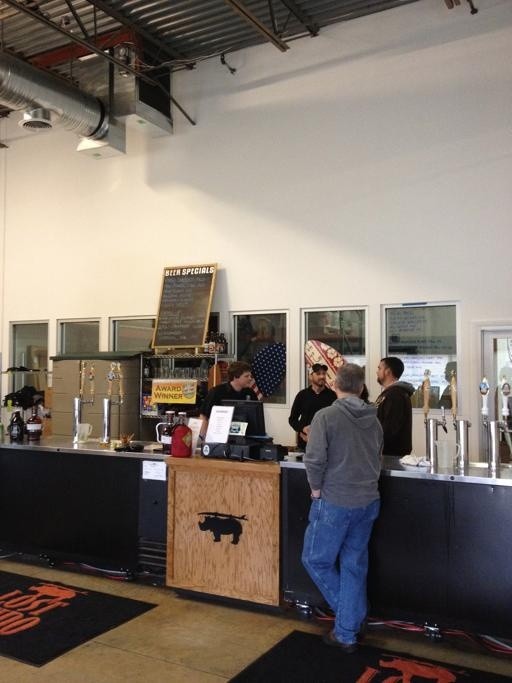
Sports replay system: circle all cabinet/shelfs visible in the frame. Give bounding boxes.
[49,358,161,443]
[139,350,234,443]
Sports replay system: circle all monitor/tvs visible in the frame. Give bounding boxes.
[221,400,266,446]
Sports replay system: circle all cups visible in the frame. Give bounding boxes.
[121,432,130,445]
[100,436,110,449]
[0,422,4,442]
[152,366,199,378]
[78,423,93,443]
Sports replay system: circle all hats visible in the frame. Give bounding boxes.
[309,363,327,374]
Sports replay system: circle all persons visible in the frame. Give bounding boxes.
[438,361,458,409]
[300,363,385,655]
[197,362,260,445]
[288,364,338,453]
[373,357,415,463]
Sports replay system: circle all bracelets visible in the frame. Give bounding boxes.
[198,434,204,442]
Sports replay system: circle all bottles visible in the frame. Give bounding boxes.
[203,329,227,354]
[200,358,208,377]
[41,412,52,437]
[27,399,42,442]
[197,413,208,447]
[9,409,24,443]
[162,409,177,454]
[170,411,192,458]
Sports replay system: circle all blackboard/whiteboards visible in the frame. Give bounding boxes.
[151,263,218,349]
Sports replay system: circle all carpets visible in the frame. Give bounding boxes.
[222,625,511,681]
[0,568,158,669]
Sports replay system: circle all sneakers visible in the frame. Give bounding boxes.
[324,631,358,654]
